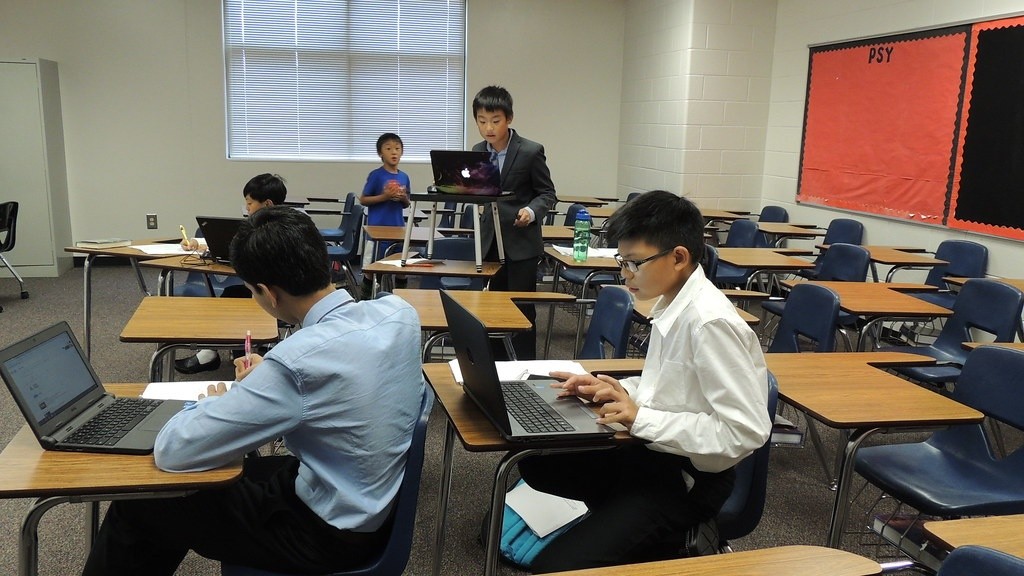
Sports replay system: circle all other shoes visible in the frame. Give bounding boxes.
[175,354,220,374]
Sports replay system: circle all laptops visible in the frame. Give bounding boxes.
[0,322,198,454]
[439,288,617,443]
[430,149,515,197]
[196,216,247,266]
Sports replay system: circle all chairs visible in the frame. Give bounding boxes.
[0,196,1024,574]
[0,200,28,313]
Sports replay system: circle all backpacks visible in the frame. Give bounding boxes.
[476,477,589,570]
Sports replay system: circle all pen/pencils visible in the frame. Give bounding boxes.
[244,330,251,370]
[406,264,434,267]
[180,225,190,246]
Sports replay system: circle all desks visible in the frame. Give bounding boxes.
[0,380,246,570]
[63,240,195,363]
[753,221,828,248]
[400,192,505,272]
[361,251,504,300]
[712,247,818,292]
[118,295,277,381]
[542,246,630,357]
[390,289,577,361]
[776,276,953,348]
[420,357,651,570]
[360,224,475,262]
[585,206,619,227]
[540,224,603,247]
[815,241,948,284]
[552,195,616,224]
[752,351,990,548]
[137,253,245,297]
[939,277,1024,344]
[690,205,754,243]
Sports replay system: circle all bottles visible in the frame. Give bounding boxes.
[572,209,591,262]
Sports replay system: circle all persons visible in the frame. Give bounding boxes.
[174,172,287,375]
[81,204,422,576]
[472,85,556,362]
[527,190,773,575]
[359,133,412,297]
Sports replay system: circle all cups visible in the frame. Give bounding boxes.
[397,184,406,196]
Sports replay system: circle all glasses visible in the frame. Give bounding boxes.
[614,245,693,272]
